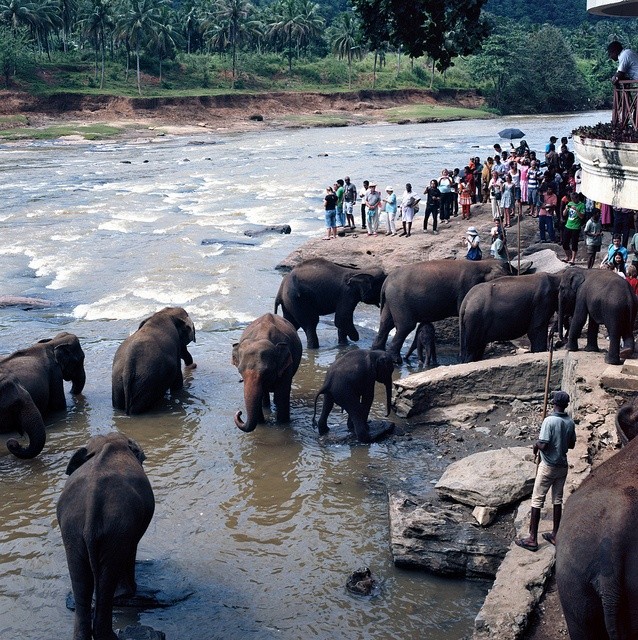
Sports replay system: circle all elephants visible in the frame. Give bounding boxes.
[404,323,437,368]
[557,267,636,365]
[312,349,394,443]
[458,269,568,363]
[55,432,155,640]
[231,313,302,432]
[372,259,533,364]
[112,306,196,416]
[0,364,46,459]
[274,257,386,349]
[0,332,86,417]
[555,394,638,640]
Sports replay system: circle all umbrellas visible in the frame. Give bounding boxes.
[497,128,525,143]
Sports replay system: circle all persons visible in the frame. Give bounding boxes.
[612,206,634,248]
[336,179,345,227]
[506,161,521,221]
[583,198,594,228]
[436,168,453,224]
[600,234,627,274]
[359,180,371,229]
[584,208,603,268]
[490,217,507,259]
[560,192,585,264]
[514,392,576,552]
[488,171,504,222]
[607,41,638,85]
[399,183,421,237]
[459,176,472,219]
[472,157,483,204]
[525,160,543,218]
[609,252,626,278]
[519,157,530,203]
[543,136,576,185]
[464,166,474,192]
[469,158,476,172]
[448,170,453,185]
[493,140,536,161]
[538,185,558,243]
[365,183,380,235]
[600,203,611,230]
[383,186,397,236]
[491,155,505,180]
[343,176,357,230]
[626,265,638,299]
[461,227,482,260]
[558,186,572,245]
[483,157,494,203]
[334,184,338,192]
[502,175,516,228]
[449,168,460,217]
[423,180,442,235]
[322,186,338,239]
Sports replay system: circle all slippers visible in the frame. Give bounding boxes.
[515,539,538,551]
[541,532,556,545]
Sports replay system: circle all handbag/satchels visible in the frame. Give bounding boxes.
[367,210,375,217]
[496,193,501,200]
[467,247,482,261]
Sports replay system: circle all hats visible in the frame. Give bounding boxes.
[553,392,570,407]
[368,182,376,187]
[467,227,478,236]
[386,186,394,191]
